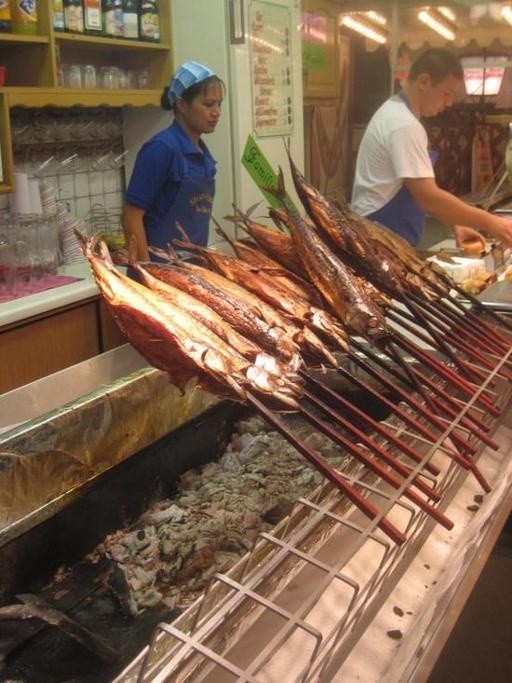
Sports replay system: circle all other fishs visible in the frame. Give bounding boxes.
[70,131,453,412]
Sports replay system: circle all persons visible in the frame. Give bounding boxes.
[344,43,512,255]
[113,59,231,284]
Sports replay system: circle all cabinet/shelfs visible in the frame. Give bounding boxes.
[0,295,115,416]
[0,0,174,194]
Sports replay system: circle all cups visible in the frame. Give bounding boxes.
[0,207,67,302]
[53,60,155,91]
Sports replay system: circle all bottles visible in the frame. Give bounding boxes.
[0,0,164,44]
